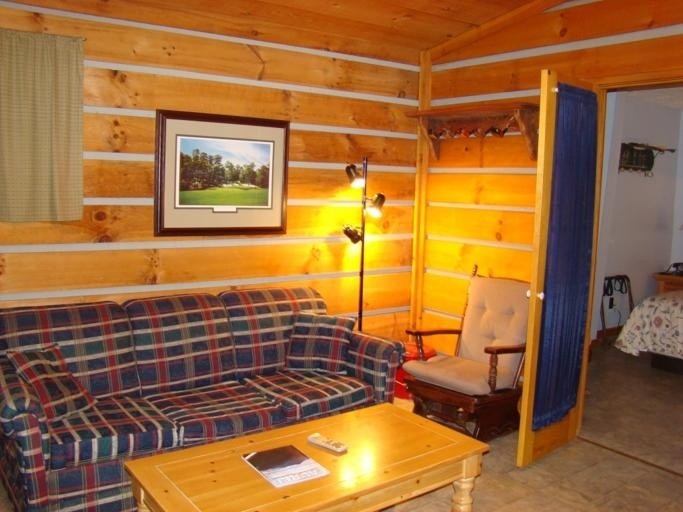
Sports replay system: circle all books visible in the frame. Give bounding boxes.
[241,445,331,489]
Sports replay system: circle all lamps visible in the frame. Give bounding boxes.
[343,155,387,334]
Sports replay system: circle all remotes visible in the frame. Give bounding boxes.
[307,432,348,453]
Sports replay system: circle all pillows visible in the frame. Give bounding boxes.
[285,313,356,375]
[4,344,99,423]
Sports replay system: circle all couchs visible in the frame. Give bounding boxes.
[1,286,405,511]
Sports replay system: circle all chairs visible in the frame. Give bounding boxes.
[400,265,530,444]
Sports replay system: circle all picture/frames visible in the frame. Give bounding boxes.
[154,108,289,234]
